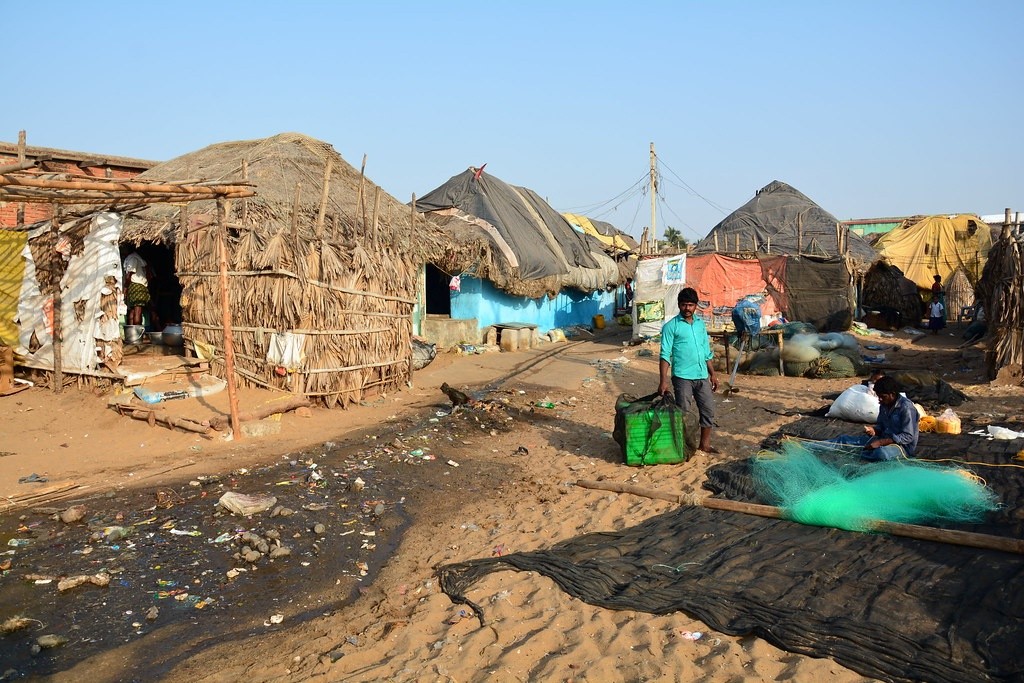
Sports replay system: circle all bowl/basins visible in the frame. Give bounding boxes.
[146,332,164,345]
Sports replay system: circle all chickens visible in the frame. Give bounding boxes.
[439,382,473,409]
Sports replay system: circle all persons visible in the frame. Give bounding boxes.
[931,275,948,328]
[927,296,944,335]
[657,288,722,456]
[777,381,918,461]
[123,244,151,346]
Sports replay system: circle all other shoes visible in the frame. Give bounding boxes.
[698,445,719,454]
[142,337,151,343]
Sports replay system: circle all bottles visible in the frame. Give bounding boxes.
[162,323,184,346]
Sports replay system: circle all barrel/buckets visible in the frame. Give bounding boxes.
[124,325,146,345]
[593,314,605,328]
